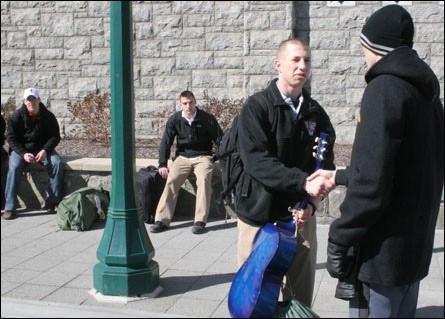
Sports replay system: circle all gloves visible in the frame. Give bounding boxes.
[335,243,363,301]
[325,240,353,278]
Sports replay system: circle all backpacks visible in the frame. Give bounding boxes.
[212,91,276,214]
[134,166,165,224]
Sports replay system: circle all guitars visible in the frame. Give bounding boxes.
[227,133,329,318]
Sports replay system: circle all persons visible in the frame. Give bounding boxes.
[150,90,224,235]
[236,38,338,317]
[306,4,444,316]
[3,87,63,220]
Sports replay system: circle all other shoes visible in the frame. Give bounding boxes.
[45,203,55,214]
[150,221,168,233]
[2,210,15,220]
[192,221,207,234]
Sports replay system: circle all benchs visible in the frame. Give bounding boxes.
[27,157,174,171]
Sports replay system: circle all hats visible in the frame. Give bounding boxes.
[24,88,41,101]
[360,4,416,56]
